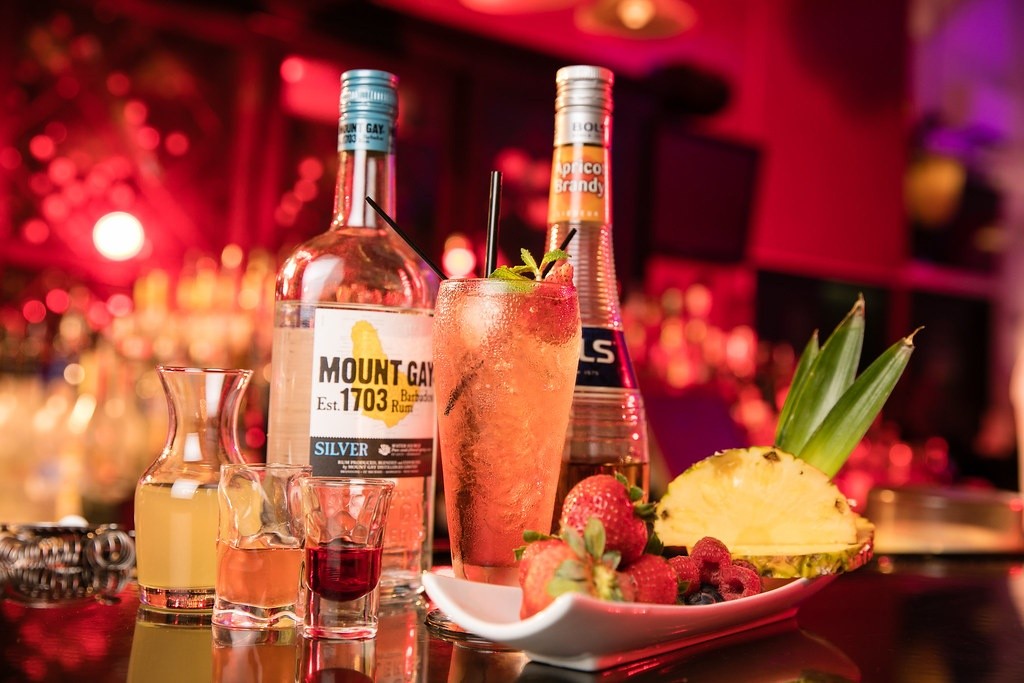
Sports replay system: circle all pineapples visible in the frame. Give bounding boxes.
[654,447,873,579]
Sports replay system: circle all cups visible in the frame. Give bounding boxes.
[297,477,397,643]
[425,282,583,633]
[212,462,315,629]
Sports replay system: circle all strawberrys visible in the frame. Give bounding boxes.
[515,263,578,343]
[520,474,761,621]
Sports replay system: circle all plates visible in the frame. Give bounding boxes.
[422,570,833,674]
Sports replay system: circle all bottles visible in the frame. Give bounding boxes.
[265,68,436,602]
[135,364,267,625]
[544,64,649,542]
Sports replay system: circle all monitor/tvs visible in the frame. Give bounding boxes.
[648,131,763,265]
